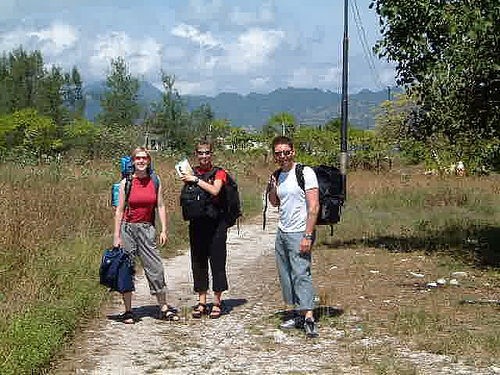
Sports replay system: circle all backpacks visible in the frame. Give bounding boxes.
[111,156,160,208]
[270,164,344,225]
[179,167,221,222]
[219,172,242,228]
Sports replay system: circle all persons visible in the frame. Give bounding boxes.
[269,136,320,337]
[179,141,226,319]
[114,146,180,324]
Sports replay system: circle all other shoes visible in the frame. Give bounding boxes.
[280,315,305,329]
[304,317,319,337]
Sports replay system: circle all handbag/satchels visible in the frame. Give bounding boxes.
[98,244,137,293]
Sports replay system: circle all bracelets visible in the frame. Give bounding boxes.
[303,233,314,240]
[195,176,199,183]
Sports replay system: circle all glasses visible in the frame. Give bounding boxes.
[134,156,149,161]
[197,151,211,155]
[275,150,292,156]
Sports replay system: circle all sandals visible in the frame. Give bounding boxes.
[154,305,180,321]
[120,310,136,324]
[209,302,226,319]
[192,302,207,318]
